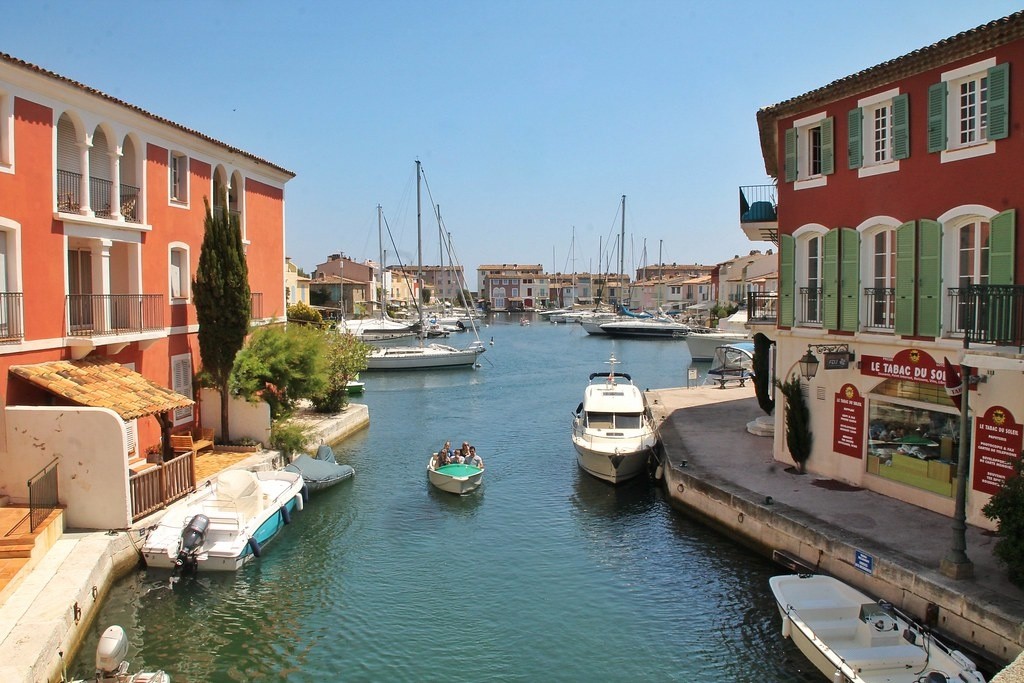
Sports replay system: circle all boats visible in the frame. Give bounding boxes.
[344,380,366,394]
[572,352,658,486]
[769,572,987,683]
[142,467,309,585]
[277,446,355,495]
[684,291,778,364]
[701,342,755,385]
[427,452,484,496]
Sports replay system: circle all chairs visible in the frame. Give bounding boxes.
[56,192,80,214]
[95,194,138,222]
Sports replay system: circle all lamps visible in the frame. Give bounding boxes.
[800,342,853,381]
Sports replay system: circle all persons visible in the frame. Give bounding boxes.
[519,317,530,325]
[431,441,484,474]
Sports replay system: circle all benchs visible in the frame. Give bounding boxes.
[713,368,750,388]
[837,644,927,679]
[170,427,215,459]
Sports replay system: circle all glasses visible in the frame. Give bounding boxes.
[470,451,475,452]
[464,445,469,446]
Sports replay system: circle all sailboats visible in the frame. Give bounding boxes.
[339,204,426,343]
[356,157,486,372]
[538,193,666,336]
[598,238,691,338]
[398,203,483,332]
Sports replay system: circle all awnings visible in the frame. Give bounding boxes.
[8,356,197,421]
